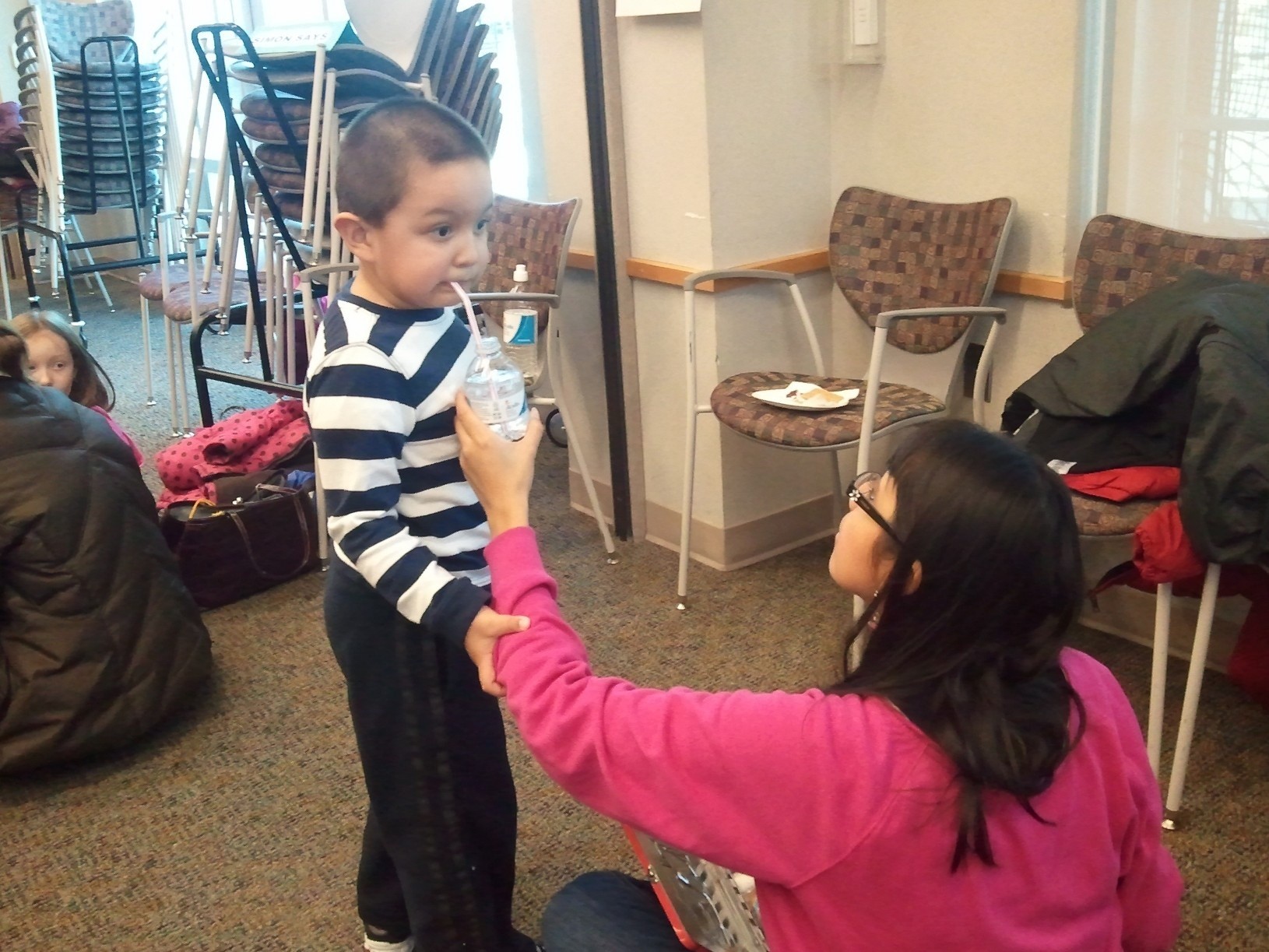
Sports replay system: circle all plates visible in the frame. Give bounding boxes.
[750,390,850,412]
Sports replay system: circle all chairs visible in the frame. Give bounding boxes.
[299,196,620,572]
[0,0,502,428]
[1019,214,1269,830]
[676,186,1018,672]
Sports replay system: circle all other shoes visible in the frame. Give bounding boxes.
[447,922,543,952]
[362,915,415,952]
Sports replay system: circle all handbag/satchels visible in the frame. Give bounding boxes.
[154,468,315,611]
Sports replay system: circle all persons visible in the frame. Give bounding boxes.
[298,95,551,951]
[451,380,1184,952]
[0,311,216,776]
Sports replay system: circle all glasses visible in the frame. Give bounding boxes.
[846,471,915,559]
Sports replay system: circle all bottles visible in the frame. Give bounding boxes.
[733,872,762,930]
[462,336,530,441]
[501,265,538,379]
